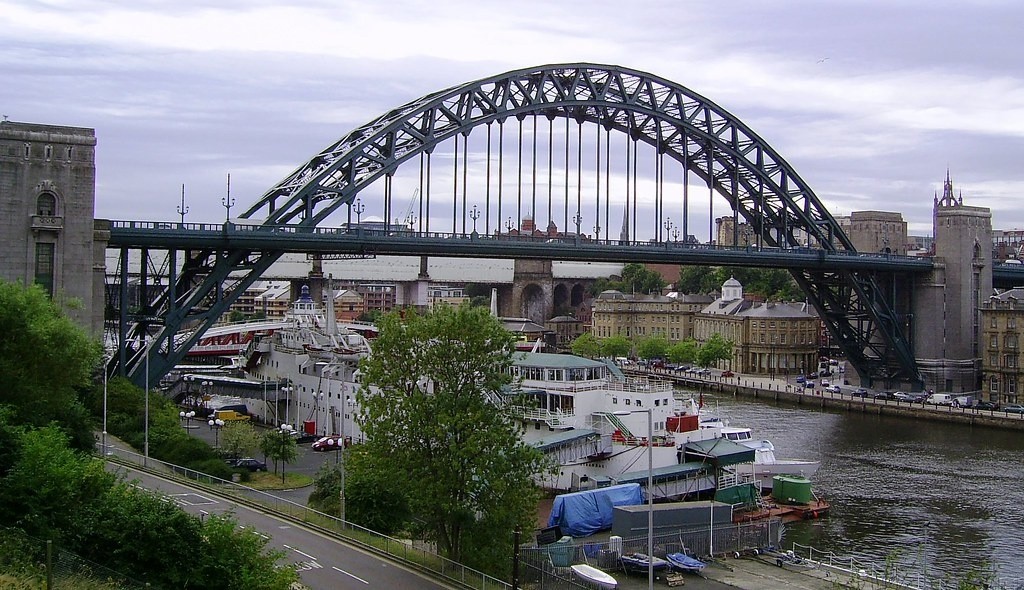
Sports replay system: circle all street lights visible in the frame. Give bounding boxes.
[179,410,196,435]
[183,376,195,412]
[315,360,345,531]
[312,392,324,435]
[280,424,292,485]
[611,408,654,589]
[208,418,225,449]
[282,387,294,423]
[202,381,213,420]
[124,337,148,470]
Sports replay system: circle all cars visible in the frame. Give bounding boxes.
[278,429,319,444]
[720,370,735,378]
[614,355,711,375]
[796,369,1024,413]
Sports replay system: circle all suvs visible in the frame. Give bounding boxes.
[311,435,347,452]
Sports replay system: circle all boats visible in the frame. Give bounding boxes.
[620,551,670,569]
[697,420,821,491]
[732,473,832,526]
[572,564,618,590]
[666,551,706,572]
